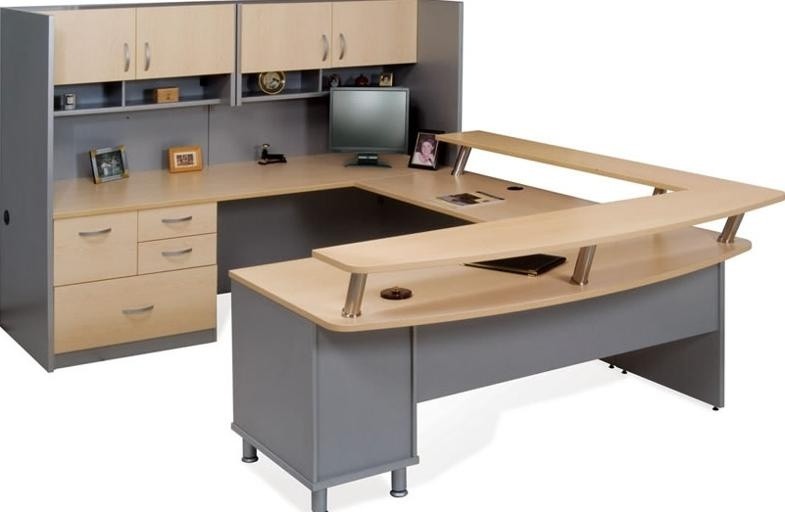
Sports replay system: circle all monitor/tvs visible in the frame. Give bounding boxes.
[328,86,410,168]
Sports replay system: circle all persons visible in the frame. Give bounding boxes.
[412,137,436,166]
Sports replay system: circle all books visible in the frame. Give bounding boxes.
[437,191,504,206]
[465,255,566,275]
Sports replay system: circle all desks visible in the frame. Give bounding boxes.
[52,151,785,512]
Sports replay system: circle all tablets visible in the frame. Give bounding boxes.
[465,253,567,277]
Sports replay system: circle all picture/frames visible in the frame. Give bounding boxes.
[410,129,447,170]
[91,142,204,183]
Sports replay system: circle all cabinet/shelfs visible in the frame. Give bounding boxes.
[3,1,237,111]
[238,4,419,102]
